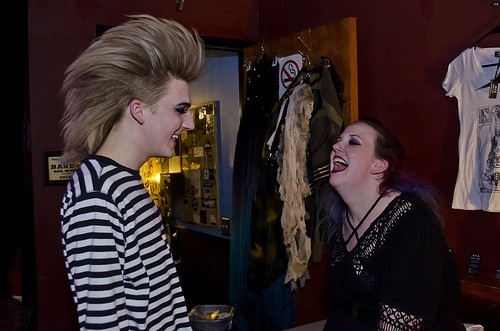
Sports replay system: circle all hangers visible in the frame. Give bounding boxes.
[446,1,499,68]
[244,43,334,108]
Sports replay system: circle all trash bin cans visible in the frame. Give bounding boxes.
[189,305,234,331]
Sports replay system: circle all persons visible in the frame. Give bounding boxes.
[62,15,205,331]
[315,118,463,331]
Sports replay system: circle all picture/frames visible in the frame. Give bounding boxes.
[41,150,83,187]
